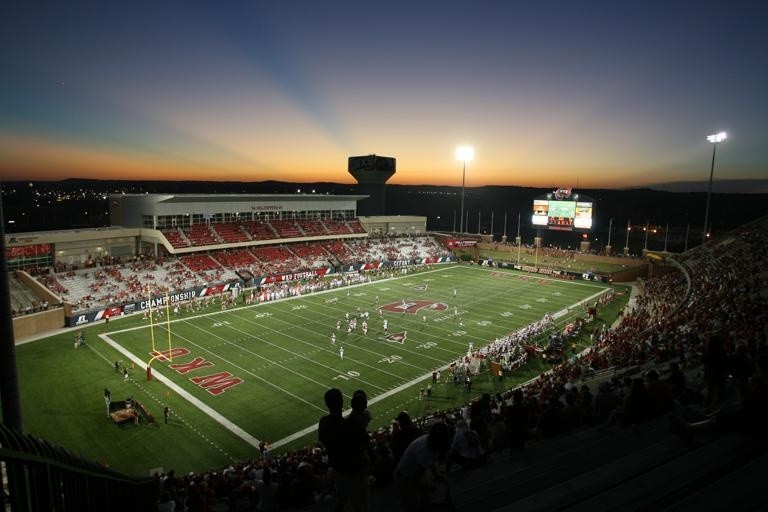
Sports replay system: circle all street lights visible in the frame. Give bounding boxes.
[454,144,472,232]
[697,129,727,241]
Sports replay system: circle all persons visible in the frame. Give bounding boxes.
[549,204,574,217]
[535,206,546,215]
[11,217,767,511]
